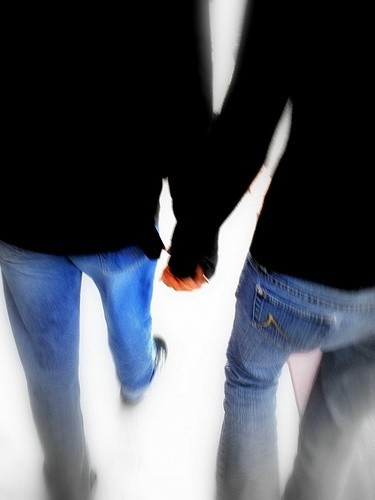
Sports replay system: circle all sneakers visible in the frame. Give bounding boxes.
[119,338,166,406]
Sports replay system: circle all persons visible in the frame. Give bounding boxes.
[1,0,218,499]
[158,0,375,499]
